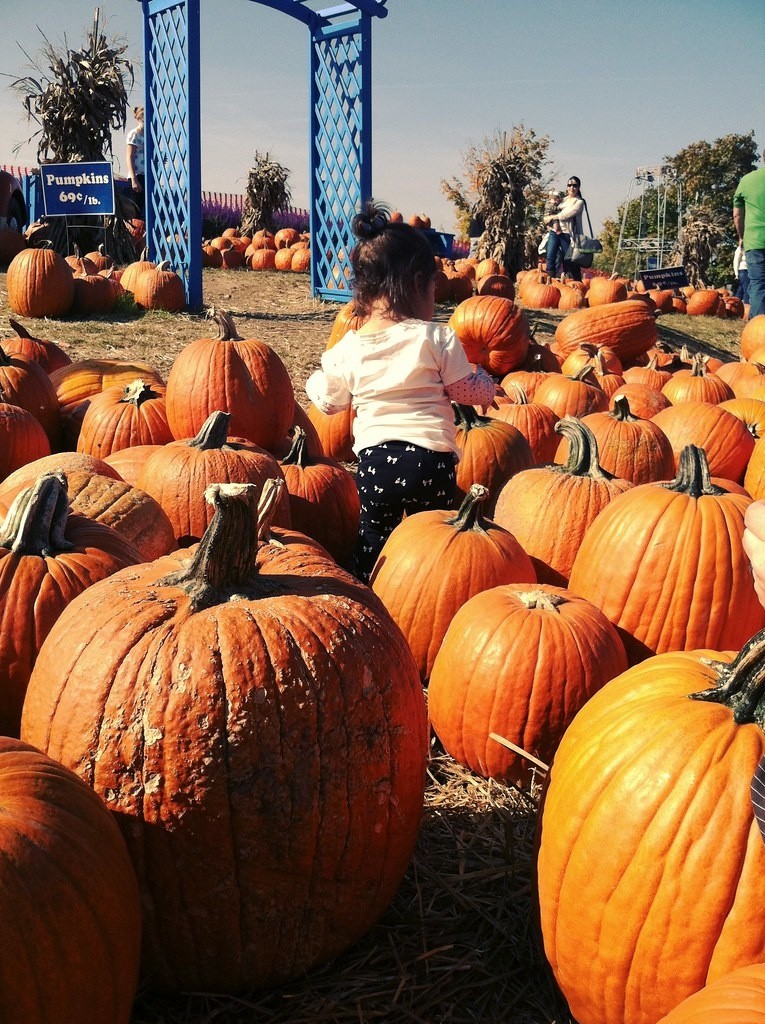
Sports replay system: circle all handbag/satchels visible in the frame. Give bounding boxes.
[563,231,602,267]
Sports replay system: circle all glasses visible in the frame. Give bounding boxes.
[567,184,577,188]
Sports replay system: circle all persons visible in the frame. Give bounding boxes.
[543,176,584,281]
[733,149,765,319]
[126,105,144,211]
[306,200,497,585]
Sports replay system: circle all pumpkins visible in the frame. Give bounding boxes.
[390,208,430,230]
[0,218,765,1024]
